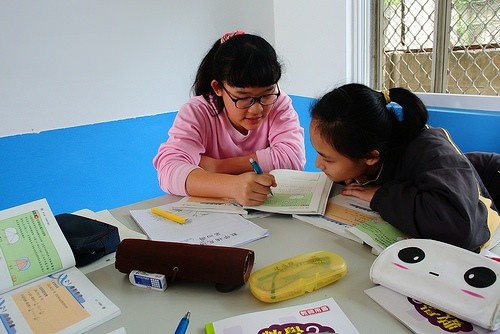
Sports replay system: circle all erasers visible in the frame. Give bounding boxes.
[129,270,167,293]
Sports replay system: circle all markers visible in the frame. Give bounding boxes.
[151,207,186,223]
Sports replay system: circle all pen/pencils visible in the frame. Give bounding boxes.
[249,158,274,196]
[174,310,191,334]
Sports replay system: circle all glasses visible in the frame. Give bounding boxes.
[222,82,280,109]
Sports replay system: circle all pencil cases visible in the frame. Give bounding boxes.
[113,238,255,293]
[368,238,500,331]
[56,213,119,267]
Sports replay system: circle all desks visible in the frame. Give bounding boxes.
[0,191,414,334]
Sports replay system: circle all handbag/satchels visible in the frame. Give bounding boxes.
[55,213,121,268]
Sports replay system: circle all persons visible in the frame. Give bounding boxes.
[153,31,306,205]
[308,81,498,254]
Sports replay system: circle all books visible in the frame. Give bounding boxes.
[363,285,500,334]
[206,297,359,334]
[172,169,334,215]
[291,193,413,255]
[0,198,117,334]
